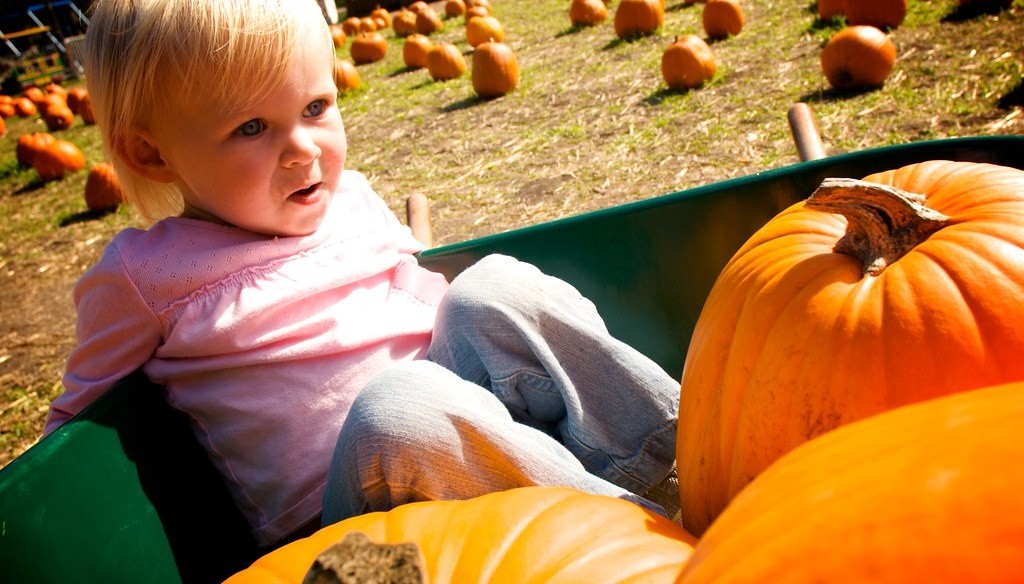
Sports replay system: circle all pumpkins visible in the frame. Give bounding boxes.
[0,0,906,210]
[219,487,696,584]
[673,381,1024,584]
[675,156,1024,535]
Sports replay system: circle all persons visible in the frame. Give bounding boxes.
[41,0,686,533]
